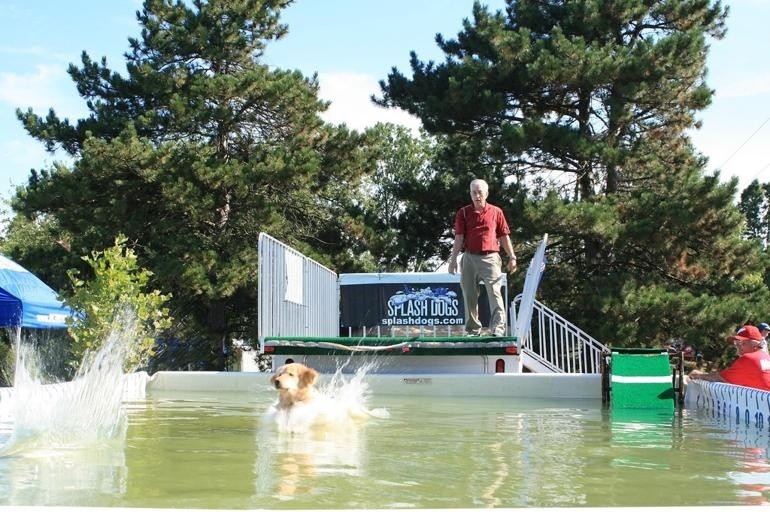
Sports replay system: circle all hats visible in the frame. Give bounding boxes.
[727,326,763,341]
[758,323,770,332]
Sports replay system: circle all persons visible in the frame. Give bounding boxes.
[448,179,517,336]
[286,359,294,364]
[687,325,770,391]
[757,323,770,354]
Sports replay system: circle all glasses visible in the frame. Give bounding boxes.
[733,340,747,346]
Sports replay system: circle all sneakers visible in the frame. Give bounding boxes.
[467,330,504,336]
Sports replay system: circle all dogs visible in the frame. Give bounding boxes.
[269,363,390,425]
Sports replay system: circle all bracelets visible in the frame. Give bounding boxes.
[510,256,518,260]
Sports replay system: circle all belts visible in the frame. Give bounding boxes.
[466,249,495,256]
